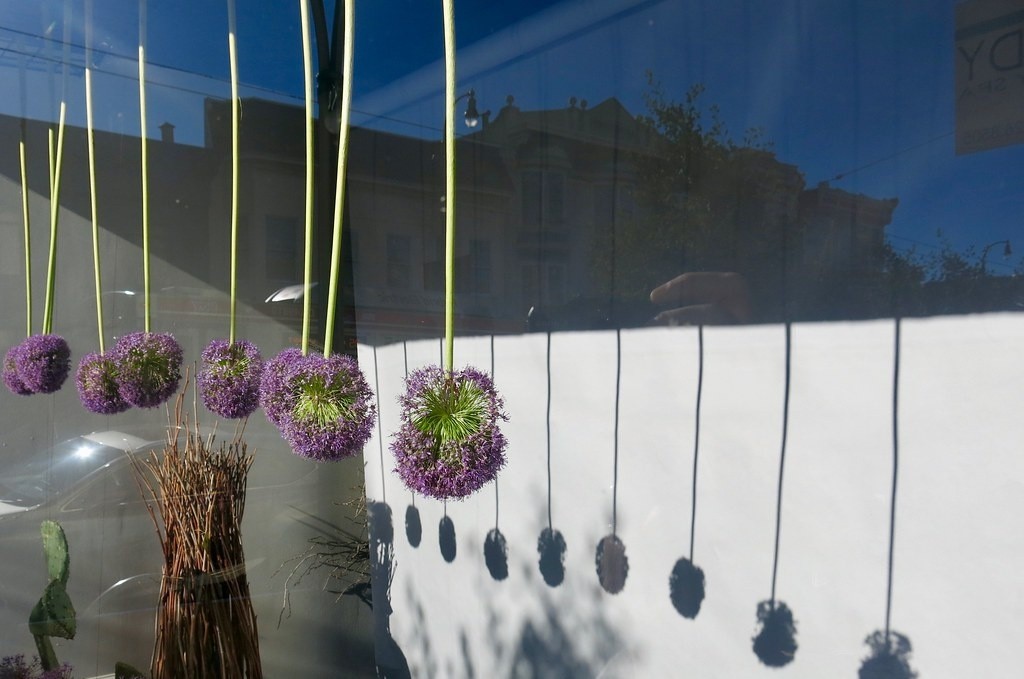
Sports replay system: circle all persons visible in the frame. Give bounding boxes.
[644,269,749,326]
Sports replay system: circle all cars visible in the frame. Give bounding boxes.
[0,421,321,561]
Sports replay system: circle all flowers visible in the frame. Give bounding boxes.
[0,335,71,397]
[196,339,263,419]
[388,363,511,502]
[259,348,378,462]
[72,350,132,416]
[106,333,185,410]
